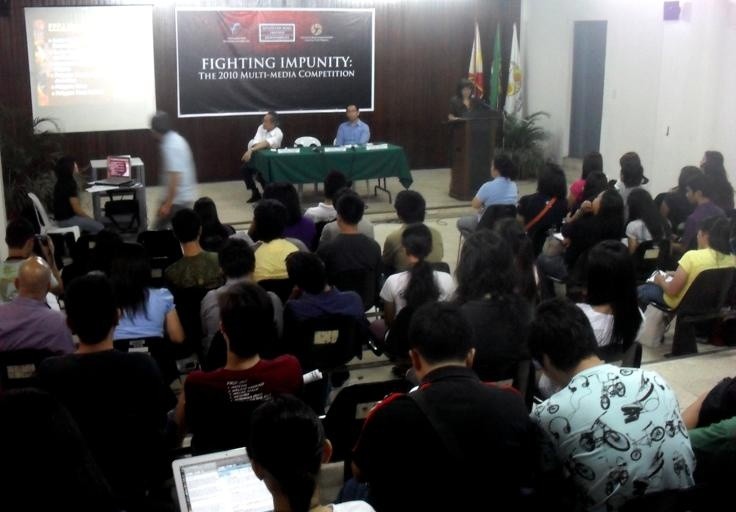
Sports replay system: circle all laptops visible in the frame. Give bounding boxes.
[95,156,133,186]
[172,446,274,512]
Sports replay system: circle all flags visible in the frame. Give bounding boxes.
[467,22,485,99]
[504,24,524,126]
[489,23,502,110]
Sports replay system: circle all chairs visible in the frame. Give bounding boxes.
[289,135,324,199]
[331,136,371,195]
[25,190,81,246]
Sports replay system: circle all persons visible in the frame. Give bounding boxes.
[177,282,303,455]
[151,111,197,229]
[449,79,496,120]
[335,302,528,511]
[36,270,180,511]
[1,151,735,407]
[335,103,370,147]
[247,394,376,511]
[681,375,736,450]
[528,298,698,511]
[53,156,104,233]
[241,110,283,203]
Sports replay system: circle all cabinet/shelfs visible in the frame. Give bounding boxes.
[88,154,149,235]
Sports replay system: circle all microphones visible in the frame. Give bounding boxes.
[471,94,493,110]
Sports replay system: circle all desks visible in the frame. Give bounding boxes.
[245,141,413,212]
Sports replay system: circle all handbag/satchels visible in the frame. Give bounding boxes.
[105,200,138,217]
[543,237,564,257]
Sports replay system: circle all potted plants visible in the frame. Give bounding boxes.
[488,100,560,180]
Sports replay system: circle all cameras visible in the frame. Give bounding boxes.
[33,233,47,246]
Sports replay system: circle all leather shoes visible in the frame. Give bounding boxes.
[247,194,260,203]
